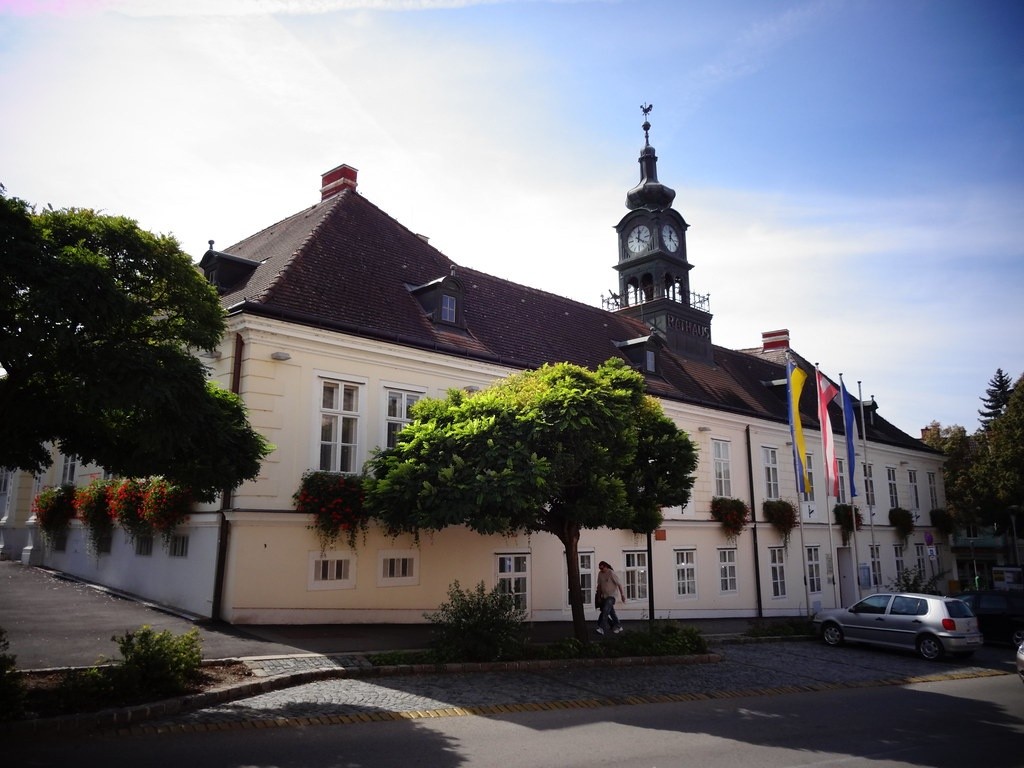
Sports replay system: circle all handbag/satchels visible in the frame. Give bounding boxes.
[595,594,600,608]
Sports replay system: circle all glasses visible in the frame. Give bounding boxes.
[599,567,604,571]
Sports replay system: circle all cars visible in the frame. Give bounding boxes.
[811,591,986,664]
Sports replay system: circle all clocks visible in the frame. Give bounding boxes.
[628,225,650,253]
[662,224,680,253]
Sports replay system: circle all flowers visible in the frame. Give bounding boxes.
[710,496,753,545]
[31,474,192,559]
[833,504,864,546]
[291,469,433,557]
[764,498,801,549]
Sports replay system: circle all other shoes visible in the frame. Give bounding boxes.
[613,626,623,633]
[596,627,604,635]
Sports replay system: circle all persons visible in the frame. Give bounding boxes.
[593,561,625,635]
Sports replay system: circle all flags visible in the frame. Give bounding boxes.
[780,358,859,498]
